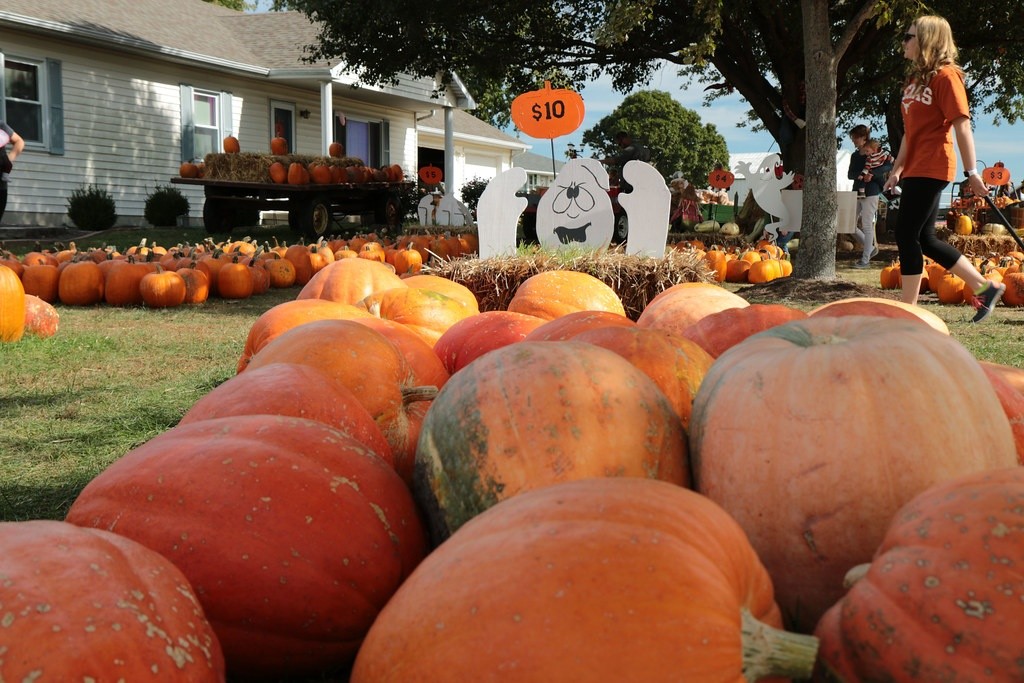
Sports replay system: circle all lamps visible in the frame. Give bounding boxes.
[300,109,311,120]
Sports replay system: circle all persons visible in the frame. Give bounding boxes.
[669,181,704,232]
[960,161,1024,207]
[0,119,25,222]
[883,15,1007,324]
[847,124,895,268]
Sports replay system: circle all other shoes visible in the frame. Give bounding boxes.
[850,260,871,269]
[869,248,877,260]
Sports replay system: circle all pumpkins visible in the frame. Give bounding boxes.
[0,133,1024,683]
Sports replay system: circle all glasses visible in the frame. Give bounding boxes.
[905,32,917,43]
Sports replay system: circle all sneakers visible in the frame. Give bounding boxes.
[968,282,1006,324]
